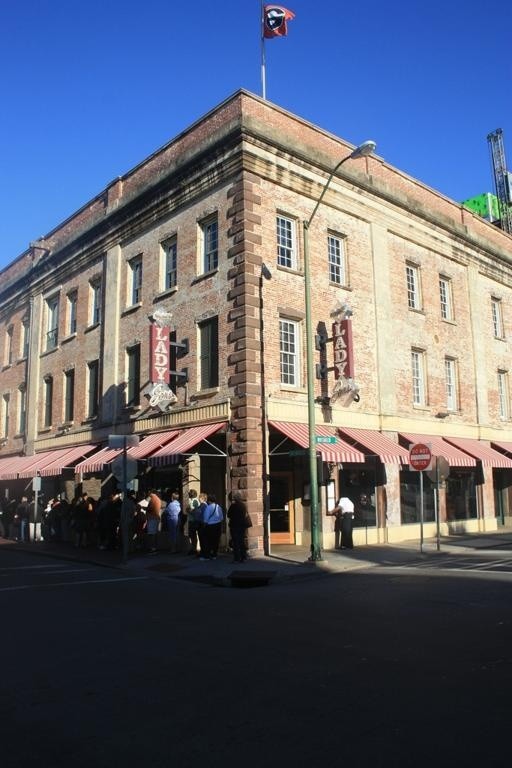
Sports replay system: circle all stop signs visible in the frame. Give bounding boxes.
[406,439,433,473]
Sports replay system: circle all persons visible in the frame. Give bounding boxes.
[227,492,252,564]
[0,487,225,561]
[326,496,355,549]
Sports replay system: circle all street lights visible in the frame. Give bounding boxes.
[299,139,377,564]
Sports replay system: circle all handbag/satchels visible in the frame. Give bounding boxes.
[245,510,252,527]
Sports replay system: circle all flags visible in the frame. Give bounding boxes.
[262,4,297,39]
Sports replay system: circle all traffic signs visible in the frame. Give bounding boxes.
[313,436,336,444]
